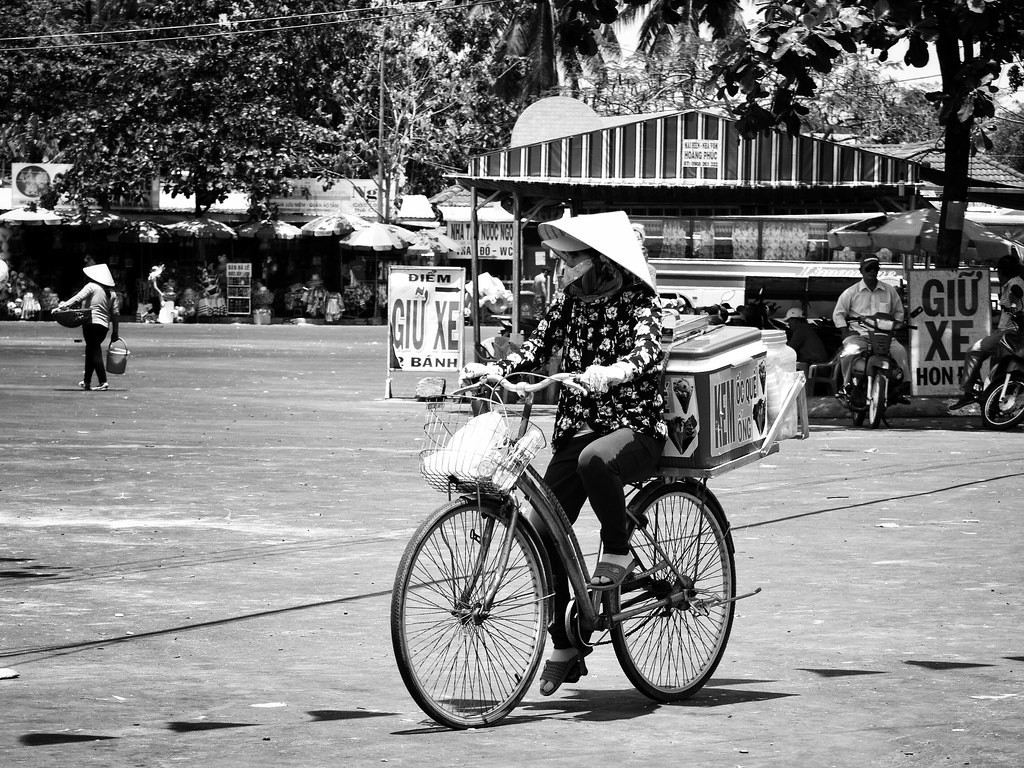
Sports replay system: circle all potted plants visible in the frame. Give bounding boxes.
[252,290,274,326]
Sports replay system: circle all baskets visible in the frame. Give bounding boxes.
[418,393,548,496]
[52,309,92,328]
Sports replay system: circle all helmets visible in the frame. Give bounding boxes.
[537,210,657,295]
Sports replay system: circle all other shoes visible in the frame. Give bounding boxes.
[91,382,108,391]
[949,393,975,410]
[79,381,91,389]
[889,393,911,404]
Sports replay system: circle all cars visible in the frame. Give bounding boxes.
[658,292,686,309]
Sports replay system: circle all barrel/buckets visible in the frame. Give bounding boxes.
[106,337,130,374]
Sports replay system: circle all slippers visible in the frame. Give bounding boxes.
[586,560,636,591]
[539,646,594,696]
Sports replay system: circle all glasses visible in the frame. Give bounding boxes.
[863,265,879,272]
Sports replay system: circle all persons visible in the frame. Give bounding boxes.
[948,257,1024,408]
[257,281,267,293]
[458,210,669,695]
[832,255,912,404]
[141,263,167,324]
[531,265,552,319]
[784,308,830,377]
[51,265,119,391]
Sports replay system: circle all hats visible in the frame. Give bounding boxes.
[540,232,591,253]
[994,254,1019,271]
[632,222,646,242]
[859,253,880,266]
[83,263,116,287]
[782,307,806,322]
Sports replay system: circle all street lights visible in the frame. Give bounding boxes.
[378,24,392,223]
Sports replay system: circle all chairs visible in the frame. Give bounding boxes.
[807,345,846,398]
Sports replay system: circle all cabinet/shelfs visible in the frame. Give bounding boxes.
[227,277,252,315]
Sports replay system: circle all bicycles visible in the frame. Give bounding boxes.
[385,362,763,730]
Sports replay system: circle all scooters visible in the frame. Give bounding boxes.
[976,283,1024,429]
[834,305,923,429]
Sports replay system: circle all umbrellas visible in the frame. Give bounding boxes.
[0,207,462,257]
[827,208,1024,267]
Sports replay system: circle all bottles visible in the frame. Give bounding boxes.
[491,425,546,490]
[760,329,799,440]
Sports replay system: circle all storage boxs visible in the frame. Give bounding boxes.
[656,324,769,469]
